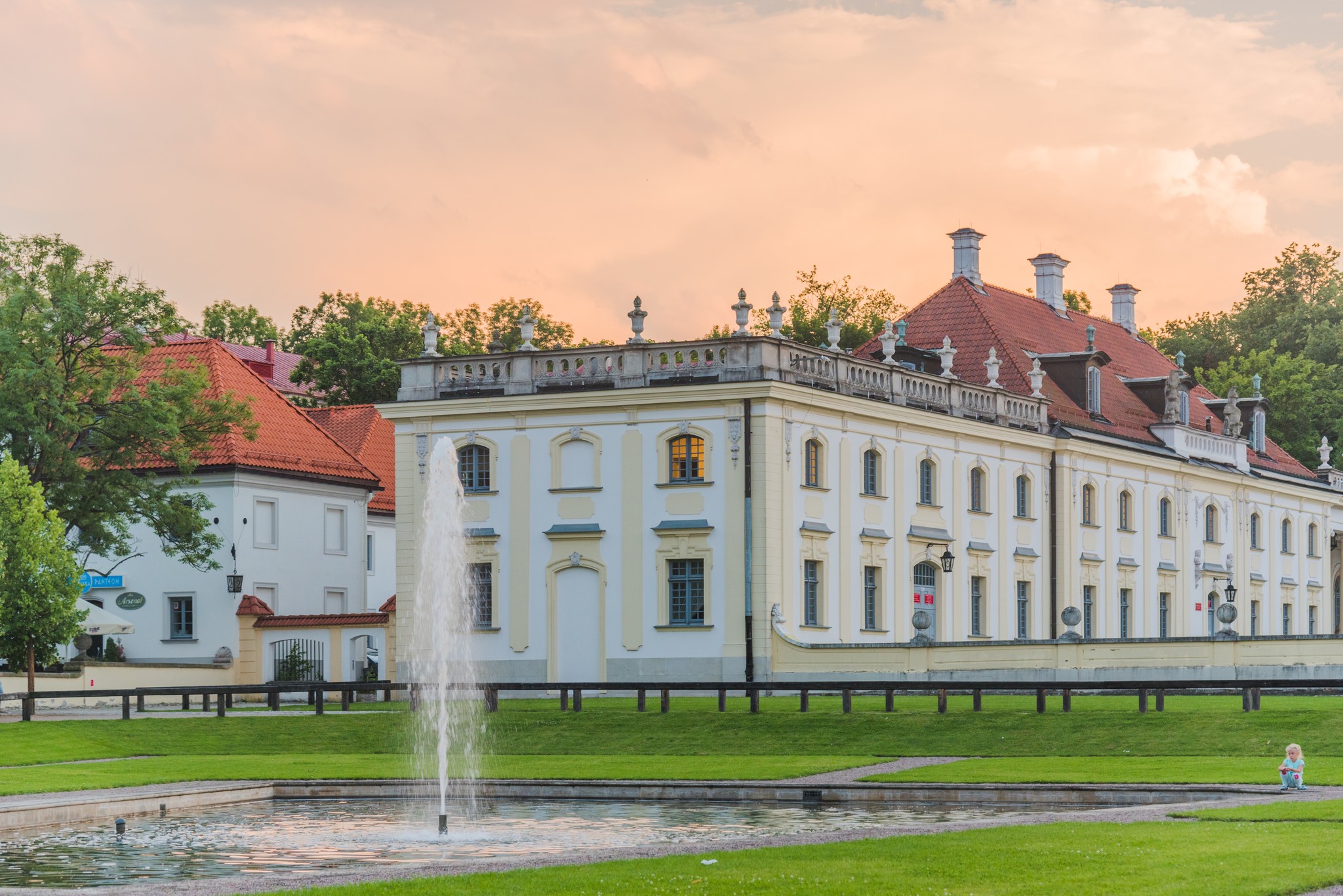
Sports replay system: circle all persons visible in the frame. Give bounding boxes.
[1278,743,1308,791]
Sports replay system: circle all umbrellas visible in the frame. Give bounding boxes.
[40,594,135,662]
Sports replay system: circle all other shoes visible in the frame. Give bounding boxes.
[1298,785,1308,789]
[1280,785,1288,790]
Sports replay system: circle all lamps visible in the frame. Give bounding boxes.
[214,517,246,599]
[927,542,955,574]
[1214,577,1237,602]
